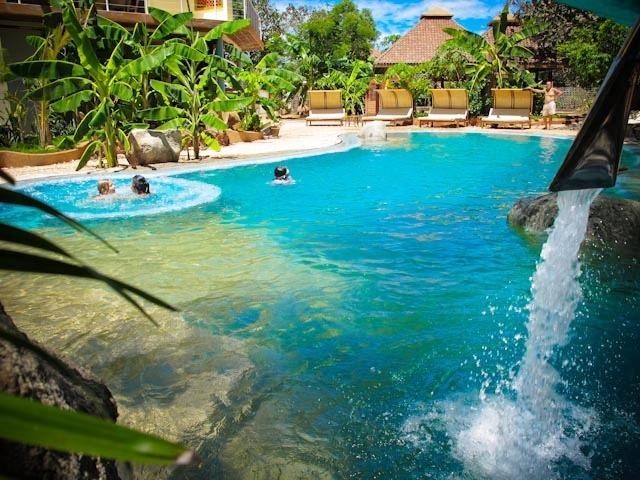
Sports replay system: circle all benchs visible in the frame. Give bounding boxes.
[305,87,533,130]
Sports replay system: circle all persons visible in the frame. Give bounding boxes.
[124,173,151,201]
[272,164,292,184]
[525,80,565,130]
[90,179,119,201]
